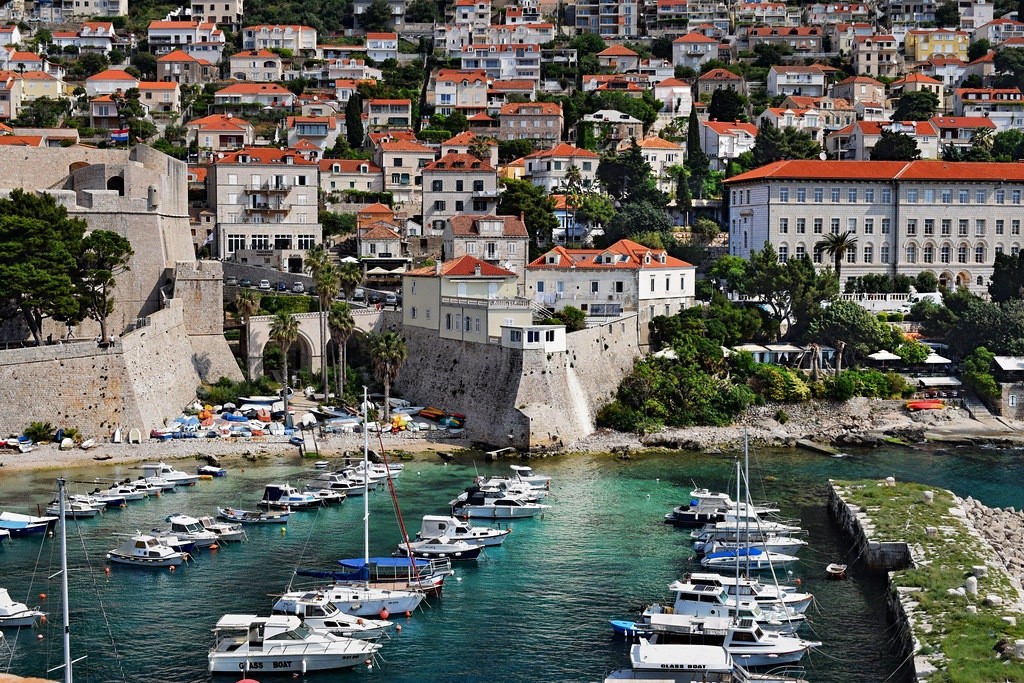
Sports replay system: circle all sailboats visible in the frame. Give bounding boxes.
[0,476,130,683]
[269,384,428,616]
[607,461,823,668]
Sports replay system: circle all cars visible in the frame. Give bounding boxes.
[225,274,398,306]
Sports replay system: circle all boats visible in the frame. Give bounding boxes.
[196,514,247,544]
[0,529,11,542]
[67,460,215,513]
[336,557,453,577]
[148,512,221,550]
[151,383,467,445]
[305,458,405,497]
[45,499,101,519]
[0,511,59,533]
[295,591,394,644]
[197,464,228,476]
[638,423,817,637]
[258,480,322,509]
[0,586,47,629]
[205,613,383,673]
[396,532,486,562]
[0,520,48,537]
[600,637,809,683]
[105,529,196,567]
[0,428,142,452]
[450,457,553,522]
[215,503,295,524]
[415,499,511,547]
[825,562,847,580]
[300,484,349,504]
